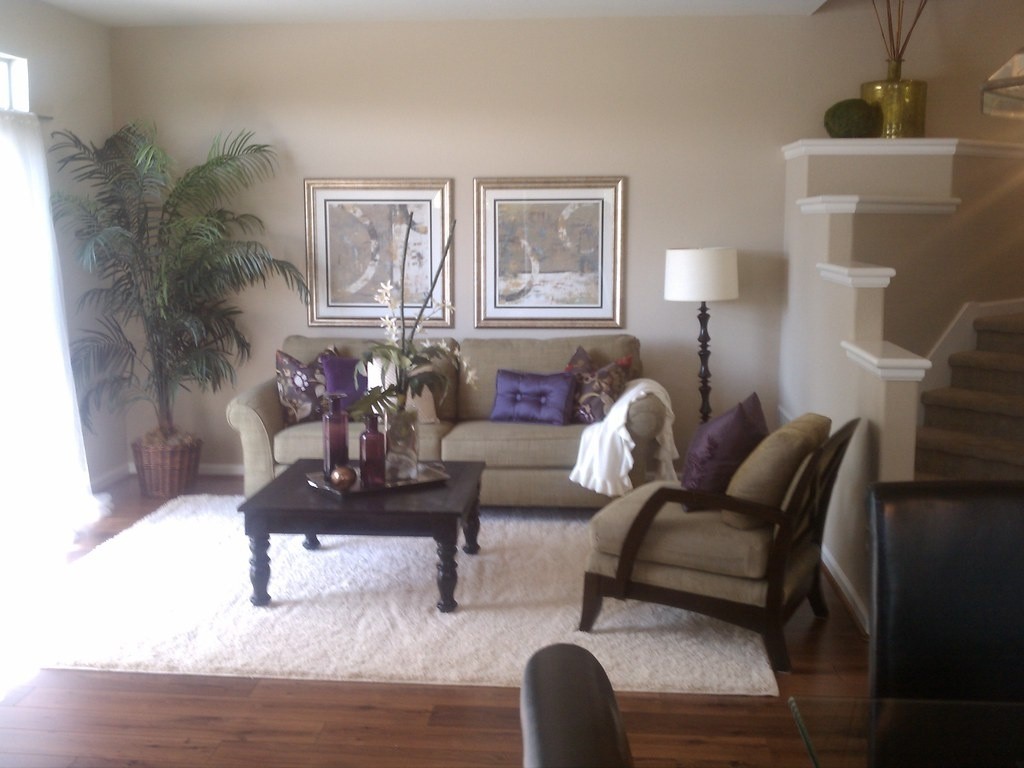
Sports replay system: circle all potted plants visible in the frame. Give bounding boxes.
[47,117,313,498]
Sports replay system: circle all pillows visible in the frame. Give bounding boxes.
[723,411,830,531]
[275,344,340,429]
[565,346,633,424]
[681,392,768,512]
[490,370,577,424]
[365,350,429,415]
[322,354,368,421]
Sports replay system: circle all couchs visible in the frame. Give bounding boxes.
[225,335,664,506]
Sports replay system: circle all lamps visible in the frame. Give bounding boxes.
[664,247,739,424]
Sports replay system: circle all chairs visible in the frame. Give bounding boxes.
[580,414,862,676]
[518,644,633,768]
[865,481,1024,702]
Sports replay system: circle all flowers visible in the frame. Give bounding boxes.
[373,279,457,399]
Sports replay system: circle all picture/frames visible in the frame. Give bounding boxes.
[473,177,627,329]
[304,177,455,327]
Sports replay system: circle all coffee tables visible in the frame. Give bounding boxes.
[238,459,484,613]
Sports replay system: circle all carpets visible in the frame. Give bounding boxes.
[24,494,780,697]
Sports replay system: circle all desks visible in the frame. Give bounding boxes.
[788,696,1024,768]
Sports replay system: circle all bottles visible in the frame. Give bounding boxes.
[360,413,385,490]
[322,393,349,480]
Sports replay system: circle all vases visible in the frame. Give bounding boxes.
[861,59,927,139]
